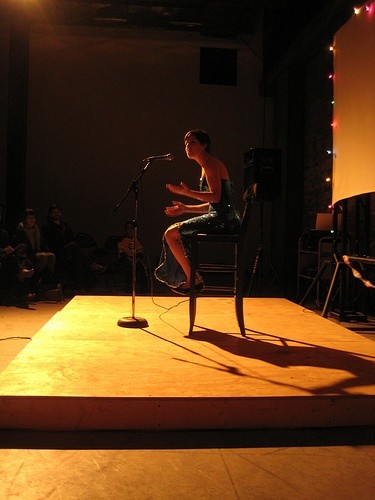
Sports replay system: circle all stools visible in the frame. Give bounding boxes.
[188,182,257,337]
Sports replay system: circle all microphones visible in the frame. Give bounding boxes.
[142,152,175,161]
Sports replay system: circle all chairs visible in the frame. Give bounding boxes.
[102,235,128,291]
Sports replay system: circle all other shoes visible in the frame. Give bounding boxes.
[27,292,36,298]
[46,288,60,295]
[18,267,35,281]
[91,262,104,271]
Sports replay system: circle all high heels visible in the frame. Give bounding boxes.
[172,281,205,295]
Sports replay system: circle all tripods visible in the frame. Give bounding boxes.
[243,198,284,297]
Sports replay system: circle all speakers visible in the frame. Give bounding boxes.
[243,148,281,197]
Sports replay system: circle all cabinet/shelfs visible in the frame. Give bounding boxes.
[298,236,343,308]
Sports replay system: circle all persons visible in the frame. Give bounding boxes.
[7,207,62,304]
[39,204,104,296]
[0,228,38,301]
[162,127,242,294]
[116,218,148,294]
